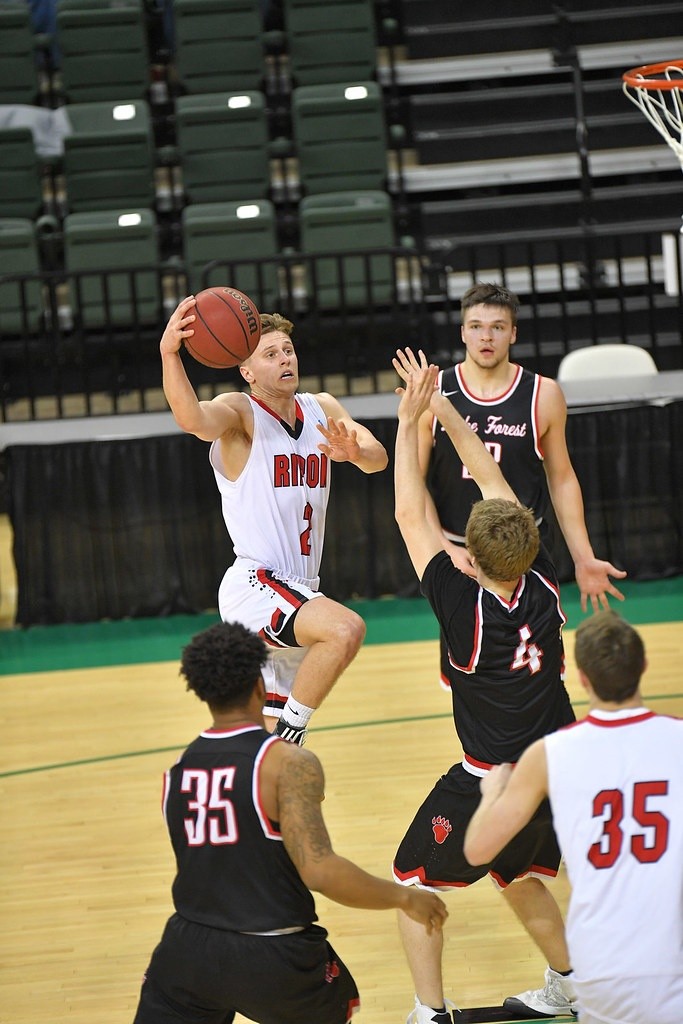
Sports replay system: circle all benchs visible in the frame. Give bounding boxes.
[383,0,683,295]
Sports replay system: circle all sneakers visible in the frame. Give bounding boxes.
[403,993,453,1024]
[503,965,581,1018]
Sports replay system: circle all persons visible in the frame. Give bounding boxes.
[417,283,628,690]
[393,348,579,1024]
[160,294,388,745]
[463,611,683,1024]
[133,622,448,1024]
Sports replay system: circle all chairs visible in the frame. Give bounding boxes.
[0,0,394,329]
[557,344,657,383]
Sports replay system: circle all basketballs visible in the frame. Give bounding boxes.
[180,286,263,369]
